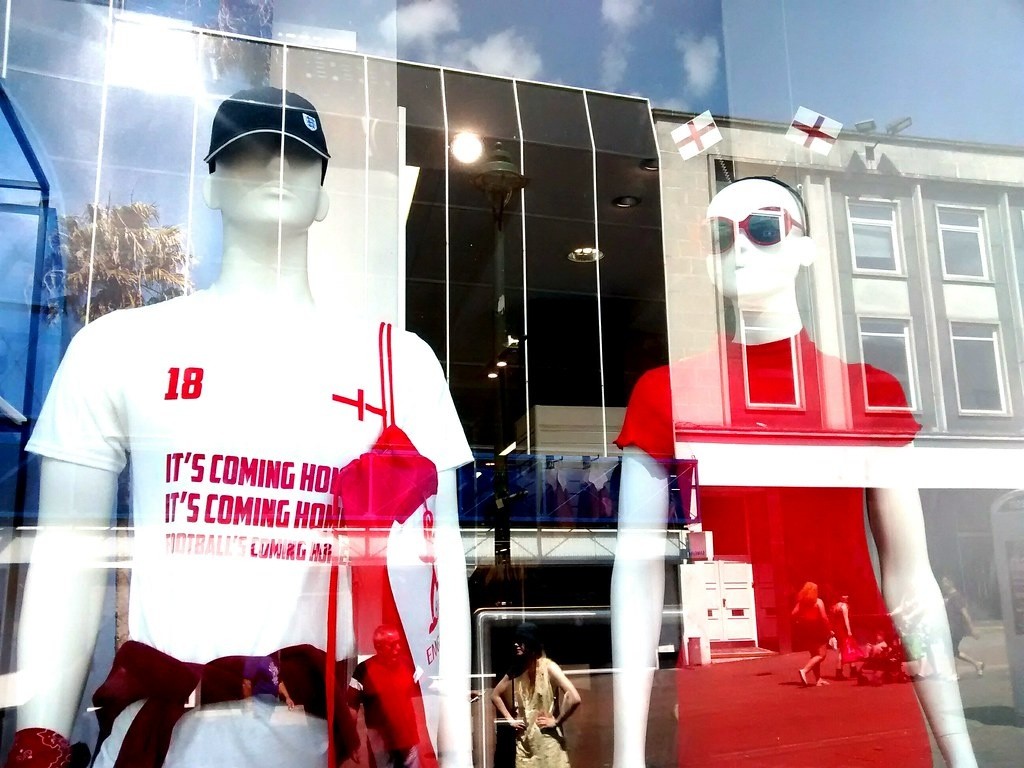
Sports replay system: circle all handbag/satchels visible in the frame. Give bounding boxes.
[840,635,866,662]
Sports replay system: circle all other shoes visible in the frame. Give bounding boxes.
[976,662,985,677]
[799,669,808,686]
[815,677,830,686]
[912,674,927,681]
[946,674,960,681]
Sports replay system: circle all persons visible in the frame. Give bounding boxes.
[607,177,984,768]
[231,615,442,768]
[789,570,989,686]
[0,86,478,768]
[490,620,583,768]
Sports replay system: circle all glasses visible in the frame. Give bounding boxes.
[691,207,805,256]
[512,639,526,647]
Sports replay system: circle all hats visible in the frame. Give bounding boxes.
[515,622,538,642]
[204,86,331,186]
